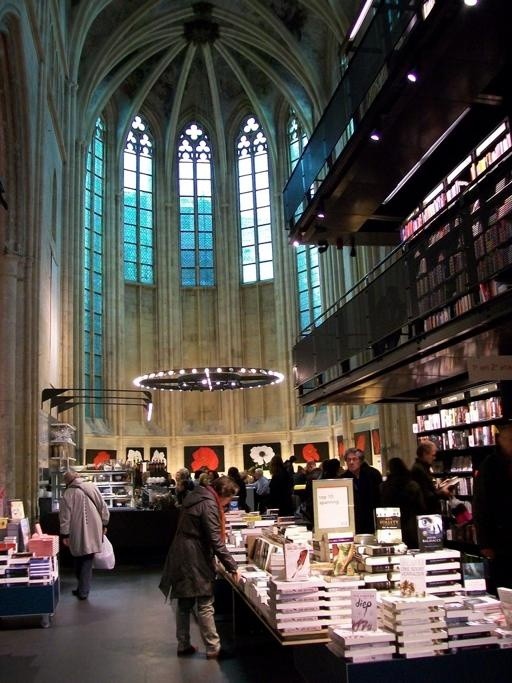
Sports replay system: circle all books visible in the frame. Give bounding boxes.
[215,396,511,661]
[397,131,511,386]
[0,517,59,588]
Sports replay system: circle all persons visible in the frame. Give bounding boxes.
[59,470,111,600]
[157,466,241,660]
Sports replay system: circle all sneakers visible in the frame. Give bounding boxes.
[72,590,86,600]
[177,645,198,657]
[207,648,238,660]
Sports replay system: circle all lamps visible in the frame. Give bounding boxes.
[131,365,283,393]
[349,243,357,256]
[317,241,329,254]
[41,387,153,420]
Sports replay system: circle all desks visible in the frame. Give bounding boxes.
[0,576,61,628]
[211,555,512,683]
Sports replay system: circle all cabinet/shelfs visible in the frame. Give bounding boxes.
[51,470,135,512]
[397,113,512,338]
[414,378,512,558]
